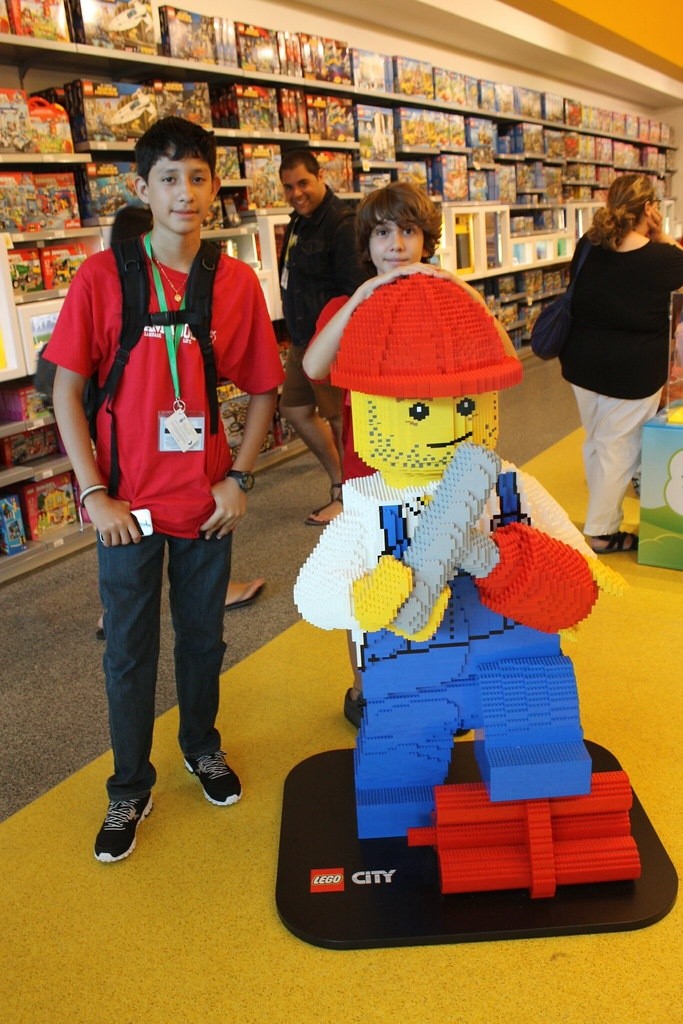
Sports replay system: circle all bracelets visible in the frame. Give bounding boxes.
[78,483,108,505]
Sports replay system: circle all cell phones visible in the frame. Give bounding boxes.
[100,508,154,543]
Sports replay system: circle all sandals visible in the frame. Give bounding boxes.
[591,531,639,553]
[305,488,345,526]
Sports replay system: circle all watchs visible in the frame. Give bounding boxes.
[224,469,255,493]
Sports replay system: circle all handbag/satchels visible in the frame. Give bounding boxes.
[529,294,575,361]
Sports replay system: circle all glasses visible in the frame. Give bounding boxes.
[652,197,662,207]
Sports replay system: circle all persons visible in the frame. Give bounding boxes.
[41,116,284,862]
[303,179,516,729]
[556,173,682,554]
[278,146,380,528]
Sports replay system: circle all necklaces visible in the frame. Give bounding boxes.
[151,251,191,302]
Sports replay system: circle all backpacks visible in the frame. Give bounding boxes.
[34,228,227,426]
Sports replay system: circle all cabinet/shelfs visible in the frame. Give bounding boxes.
[0,34,679,589]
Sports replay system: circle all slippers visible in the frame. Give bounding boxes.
[226,579,270,610]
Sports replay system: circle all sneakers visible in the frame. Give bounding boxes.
[183,746,240,806]
[91,786,152,864]
